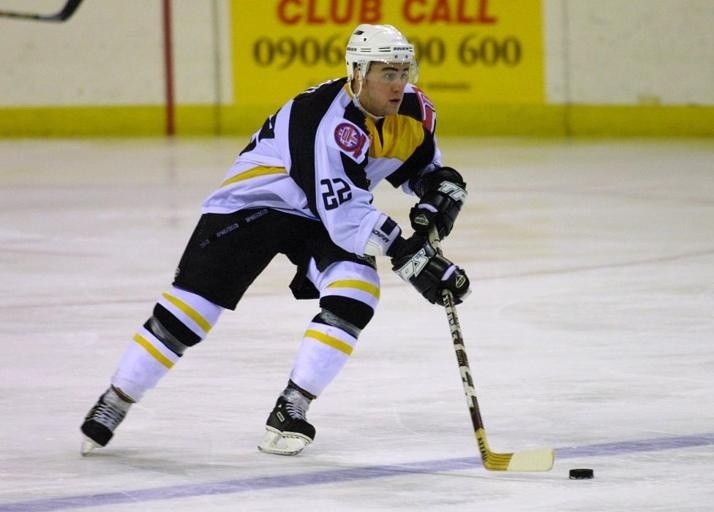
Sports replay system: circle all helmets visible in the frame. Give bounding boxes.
[344,21,421,84]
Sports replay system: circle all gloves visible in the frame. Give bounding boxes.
[390,232,472,310]
[409,165,467,240]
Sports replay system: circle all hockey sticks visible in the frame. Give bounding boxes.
[426,226,555,473]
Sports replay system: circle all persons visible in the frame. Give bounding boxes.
[82,24,469,446]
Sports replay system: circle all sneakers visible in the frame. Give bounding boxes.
[79,386,133,449]
[264,387,321,440]
